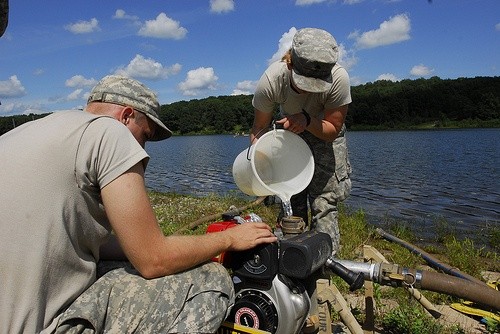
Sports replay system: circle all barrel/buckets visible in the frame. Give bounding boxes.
[233,120,315,196]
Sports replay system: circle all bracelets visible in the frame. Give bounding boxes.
[299,109,311,127]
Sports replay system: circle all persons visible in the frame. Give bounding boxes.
[250,28,354,279]
[0,75,277,334]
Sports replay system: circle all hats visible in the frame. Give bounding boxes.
[292,28,338,92]
[87,75,173,141]
